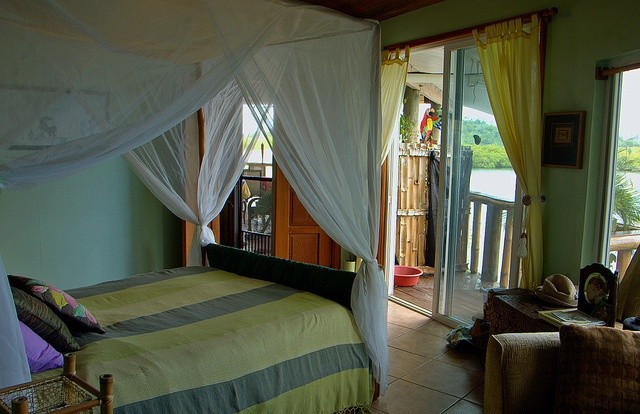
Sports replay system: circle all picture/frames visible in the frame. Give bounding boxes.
[0,83,112,152]
[540,111,587,170]
[577,262,618,329]
[536,306,606,330]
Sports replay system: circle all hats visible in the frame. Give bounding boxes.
[536,274,578,307]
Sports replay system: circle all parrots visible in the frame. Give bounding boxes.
[420,107,442,143]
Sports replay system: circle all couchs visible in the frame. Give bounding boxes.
[482,331,640,414]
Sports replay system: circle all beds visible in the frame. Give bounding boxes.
[0,243,382,414]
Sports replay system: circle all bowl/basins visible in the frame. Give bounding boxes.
[394,264,423,286]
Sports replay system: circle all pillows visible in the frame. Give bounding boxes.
[10,286,81,353]
[558,323,640,414]
[17,320,64,374]
[7,274,106,335]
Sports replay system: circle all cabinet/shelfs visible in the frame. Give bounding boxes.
[0,353,114,413]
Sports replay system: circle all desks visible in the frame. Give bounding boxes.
[489,292,626,336]
